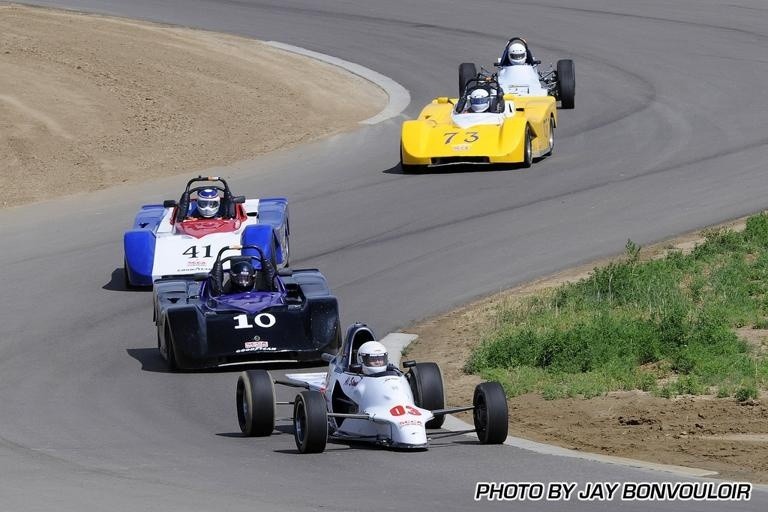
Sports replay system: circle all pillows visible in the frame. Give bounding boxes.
[508,43,527,64]
[357,341,387,375]
[197,189,221,218]
[230,261,256,291]
[470,88,490,112]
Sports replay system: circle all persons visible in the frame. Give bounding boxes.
[508,42,529,66]
[355,340,390,375]
[464,89,493,114]
[229,262,257,292]
[188,188,225,221]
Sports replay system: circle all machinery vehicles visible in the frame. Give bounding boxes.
[121,176,289,289]
[149,243,343,374]
[234,323,510,452]
[399,74,557,171]
[456,35,577,109]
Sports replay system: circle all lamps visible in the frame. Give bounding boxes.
[353,349,358,363]
[191,187,226,218]
[223,256,252,294]
